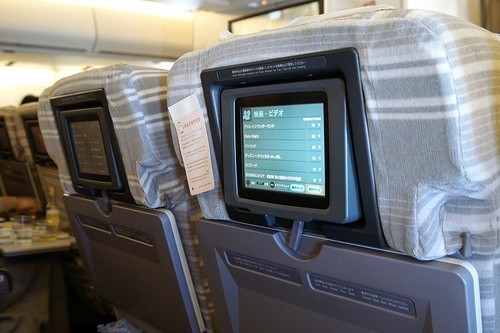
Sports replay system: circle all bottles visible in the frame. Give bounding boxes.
[46,185,60,237]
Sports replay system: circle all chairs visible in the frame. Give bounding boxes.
[0,5,500,333]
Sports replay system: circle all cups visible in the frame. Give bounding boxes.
[10,215,36,244]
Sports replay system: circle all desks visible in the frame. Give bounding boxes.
[0,217,77,257]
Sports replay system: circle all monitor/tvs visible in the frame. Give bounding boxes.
[221,79,360,224]
[60,107,123,191]
[24,120,50,158]
[0,124,12,155]
